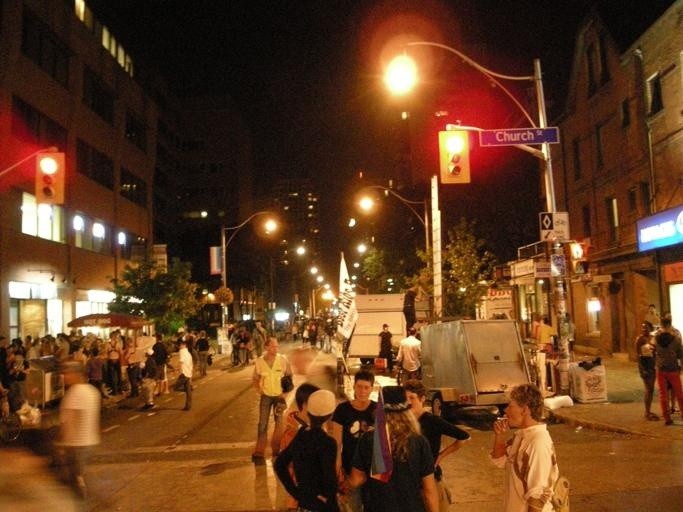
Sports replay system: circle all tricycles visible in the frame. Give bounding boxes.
[401,319,538,422]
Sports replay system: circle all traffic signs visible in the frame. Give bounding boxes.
[540,211,570,244]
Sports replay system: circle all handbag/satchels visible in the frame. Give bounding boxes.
[280,375,294,393]
[551,473,571,512]
[374,357,388,369]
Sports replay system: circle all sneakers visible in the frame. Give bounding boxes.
[139,403,155,411]
[665,418,674,424]
[670,406,680,414]
[646,414,658,421]
[251,455,266,466]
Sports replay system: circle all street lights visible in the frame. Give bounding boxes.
[218,208,276,352]
[266,236,390,340]
[353,173,444,324]
[379,40,571,397]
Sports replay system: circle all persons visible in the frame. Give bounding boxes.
[403,379,472,512]
[274,391,338,512]
[492,384,559,512]
[52,362,99,502]
[531,304,683,425]
[1,313,337,425]
[378,324,395,372]
[332,371,378,512]
[253,337,293,459]
[396,327,422,386]
[349,386,439,512]
[403,282,422,337]
[279,384,322,512]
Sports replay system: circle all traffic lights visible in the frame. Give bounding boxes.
[440,130,472,184]
[36,153,65,204]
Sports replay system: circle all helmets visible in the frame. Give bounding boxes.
[307,389,337,415]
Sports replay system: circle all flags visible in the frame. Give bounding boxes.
[337,257,357,340]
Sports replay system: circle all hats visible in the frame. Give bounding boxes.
[375,386,411,412]
[382,323,390,328]
